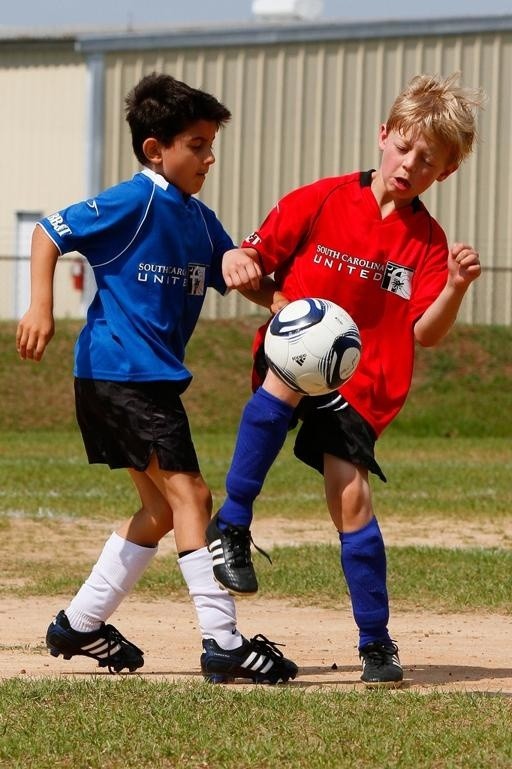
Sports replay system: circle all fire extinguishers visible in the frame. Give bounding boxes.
[70,257,84,293]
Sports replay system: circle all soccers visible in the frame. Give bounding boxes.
[263,298,362,395]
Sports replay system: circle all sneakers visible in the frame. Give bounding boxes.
[360,638,403,687]
[47,610,145,675]
[204,509,272,597]
[200,634,297,685]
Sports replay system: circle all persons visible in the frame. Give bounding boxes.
[222,73,490,690]
[13,67,297,687]
